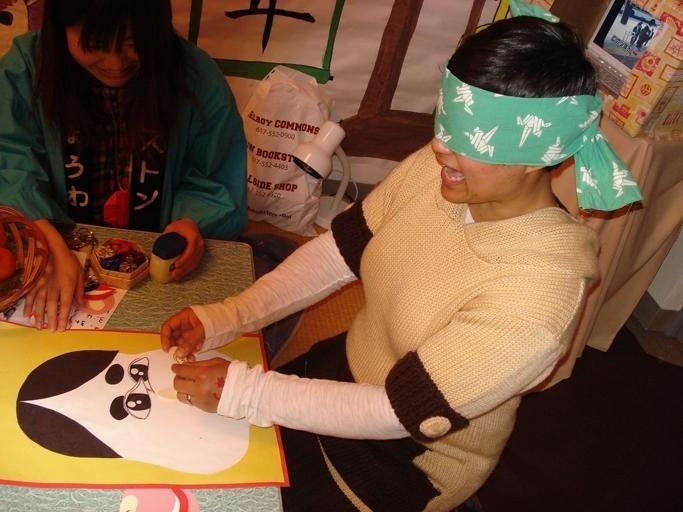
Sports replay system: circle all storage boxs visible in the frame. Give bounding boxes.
[547,0,683,138]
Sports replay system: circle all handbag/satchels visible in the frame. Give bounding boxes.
[239,64,335,237]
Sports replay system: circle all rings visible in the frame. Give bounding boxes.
[186,393,194,405]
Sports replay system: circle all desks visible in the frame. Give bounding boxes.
[1,218,286,512]
[550,114,683,387]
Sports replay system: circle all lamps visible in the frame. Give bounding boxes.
[288,120,354,230]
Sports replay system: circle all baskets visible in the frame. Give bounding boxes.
[0,205,50,313]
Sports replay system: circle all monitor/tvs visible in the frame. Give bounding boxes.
[584,0,662,95]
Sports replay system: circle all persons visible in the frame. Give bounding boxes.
[160,17,601,512]
[1,0,249,332]
